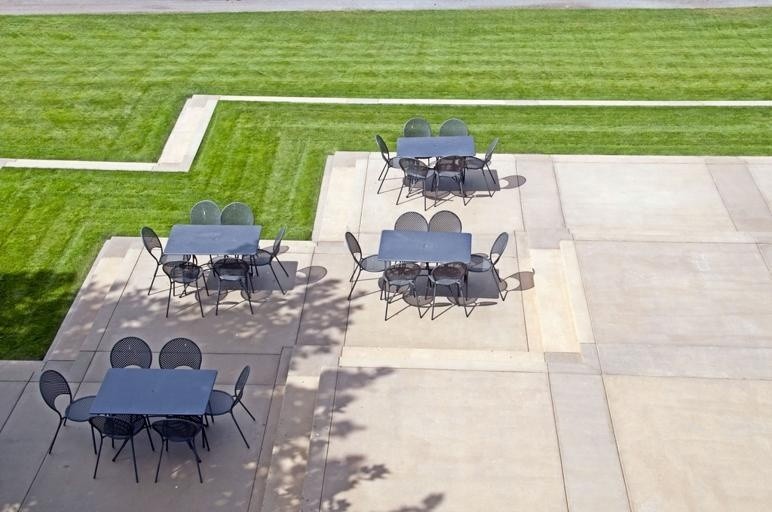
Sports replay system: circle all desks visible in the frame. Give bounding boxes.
[164,224,262,297]
[377,230,471,301]
[87,368,217,450]
[397,138,475,190]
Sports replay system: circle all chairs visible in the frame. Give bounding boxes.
[40,369,112,455]
[142,226,191,296]
[244,227,290,298]
[153,415,212,485]
[431,156,466,207]
[439,119,470,159]
[213,259,255,316]
[109,337,153,368]
[201,366,256,449]
[464,232,509,301]
[163,261,209,318]
[89,410,156,483]
[220,202,255,259]
[345,232,400,300]
[191,199,220,264]
[158,337,201,369]
[457,137,500,197]
[380,263,422,321]
[394,212,429,286]
[375,134,416,194]
[424,262,470,320]
[403,118,434,166]
[396,158,437,211]
[428,211,462,270]
